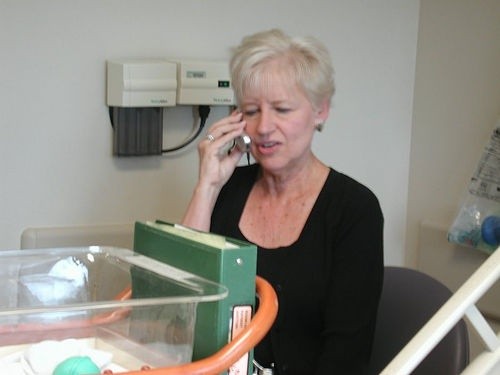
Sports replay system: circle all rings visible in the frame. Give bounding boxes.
[206,134,215,141]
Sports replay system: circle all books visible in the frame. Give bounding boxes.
[132,219,257,374]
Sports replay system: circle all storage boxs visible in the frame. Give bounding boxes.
[0,246,229,374]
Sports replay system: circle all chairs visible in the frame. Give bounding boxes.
[372,267,470,374]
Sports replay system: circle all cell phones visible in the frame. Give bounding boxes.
[234,130,252,152]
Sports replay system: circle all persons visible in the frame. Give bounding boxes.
[180,28,385,374]
